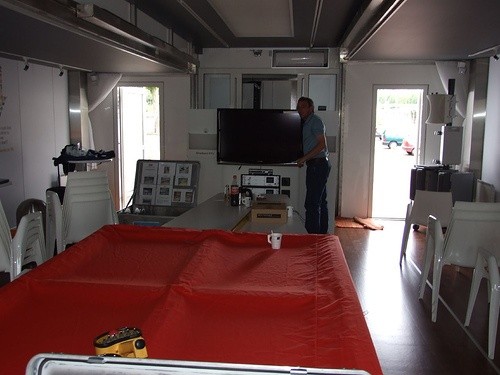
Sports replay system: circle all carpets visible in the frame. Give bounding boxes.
[336,217,385,231]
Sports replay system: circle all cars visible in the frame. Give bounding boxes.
[376,125,416,156]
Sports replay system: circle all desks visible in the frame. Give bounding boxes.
[160,193,289,233]
[66,158,113,171]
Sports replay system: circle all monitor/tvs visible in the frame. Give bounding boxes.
[217,107,304,166]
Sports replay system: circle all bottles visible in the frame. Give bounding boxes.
[231,175,239,206]
[224,185,230,201]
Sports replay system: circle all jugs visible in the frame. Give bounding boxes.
[239,186,253,205]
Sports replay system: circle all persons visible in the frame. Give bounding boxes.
[294,96,331,234]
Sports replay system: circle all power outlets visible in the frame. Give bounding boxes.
[287,206,295,217]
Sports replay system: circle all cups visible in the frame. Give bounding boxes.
[242,197,251,208]
[287,206,293,218]
[267,233,282,249]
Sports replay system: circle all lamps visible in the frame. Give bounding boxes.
[58,65,64,77]
[493,51,500,63]
[23,59,31,72]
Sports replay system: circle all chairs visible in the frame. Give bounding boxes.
[399,189,500,360]
[0,168,119,283]
